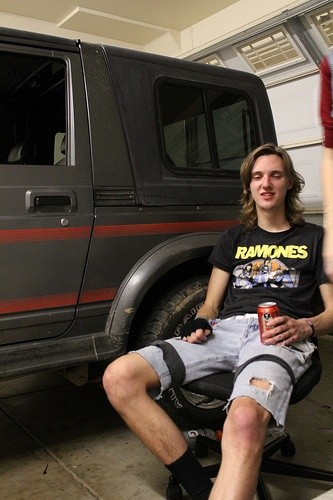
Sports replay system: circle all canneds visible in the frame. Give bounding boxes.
[257,302,280,344]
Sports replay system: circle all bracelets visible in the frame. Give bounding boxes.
[305,317,315,336]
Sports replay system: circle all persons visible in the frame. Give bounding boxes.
[103,144,333,500]
[319,53,333,283]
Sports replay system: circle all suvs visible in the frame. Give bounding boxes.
[0,24,278,430]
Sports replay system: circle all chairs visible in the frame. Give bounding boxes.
[164,335,333,500]
